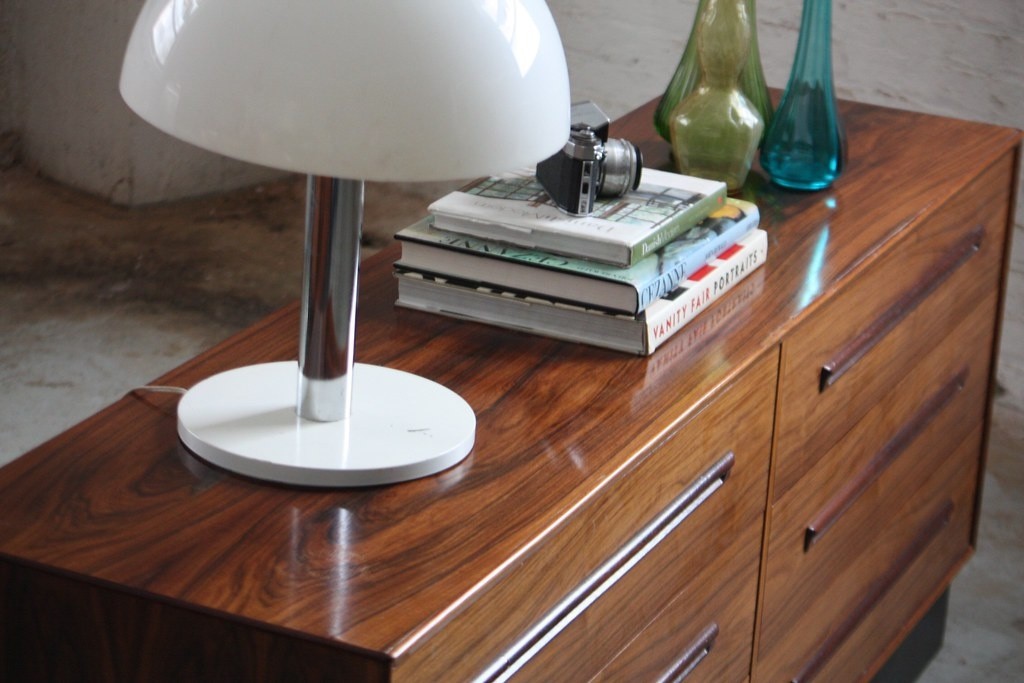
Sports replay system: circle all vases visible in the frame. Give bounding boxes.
[653,0,847,197]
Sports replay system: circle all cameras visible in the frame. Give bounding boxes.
[535,100,645,217]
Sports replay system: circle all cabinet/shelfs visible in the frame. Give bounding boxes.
[0,86,1024,683]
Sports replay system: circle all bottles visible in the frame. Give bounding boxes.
[669,0,765,196]
[759,0,849,191]
[654,0,775,149]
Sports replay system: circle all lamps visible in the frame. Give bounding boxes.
[118,0,571,488]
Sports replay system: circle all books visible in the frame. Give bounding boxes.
[392,168,769,355]
